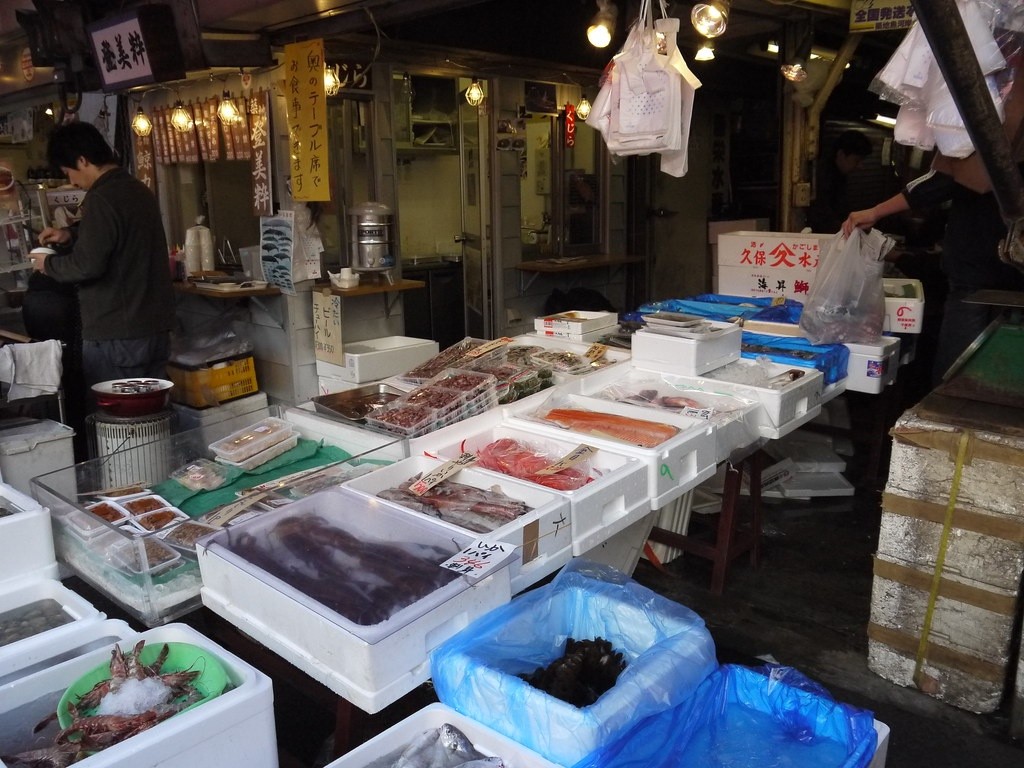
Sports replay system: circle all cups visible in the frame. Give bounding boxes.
[30,247,57,269]
[185,226,214,277]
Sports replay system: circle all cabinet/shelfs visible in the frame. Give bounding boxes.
[393,73,478,158]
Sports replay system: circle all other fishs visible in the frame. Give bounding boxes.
[391,724,503,768]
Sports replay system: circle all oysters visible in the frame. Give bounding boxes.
[0,606,65,647]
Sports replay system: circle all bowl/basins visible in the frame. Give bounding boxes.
[4,287,27,308]
[65,416,381,579]
[220,262,242,282]
[329,274,360,291]
[56,641,227,754]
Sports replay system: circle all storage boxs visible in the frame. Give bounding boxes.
[881,278,925,334]
[708,217,846,307]
[0,308,1024,768]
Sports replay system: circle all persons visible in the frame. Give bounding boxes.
[842,169,1024,399]
[26,120,177,413]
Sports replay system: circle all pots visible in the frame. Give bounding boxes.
[90,376,175,417]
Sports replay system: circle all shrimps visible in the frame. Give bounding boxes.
[0,640,204,768]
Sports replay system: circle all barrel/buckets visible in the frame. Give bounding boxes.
[95,419,172,490]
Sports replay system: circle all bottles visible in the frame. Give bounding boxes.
[111,380,159,393]
[26,162,70,188]
[167,244,186,282]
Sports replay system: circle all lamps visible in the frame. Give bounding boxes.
[690,0,734,40]
[575,85,592,121]
[465,77,483,107]
[90,61,340,137]
[693,36,716,61]
[778,13,815,83]
[584,0,618,49]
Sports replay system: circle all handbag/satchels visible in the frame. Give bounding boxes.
[798,219,886,346]
